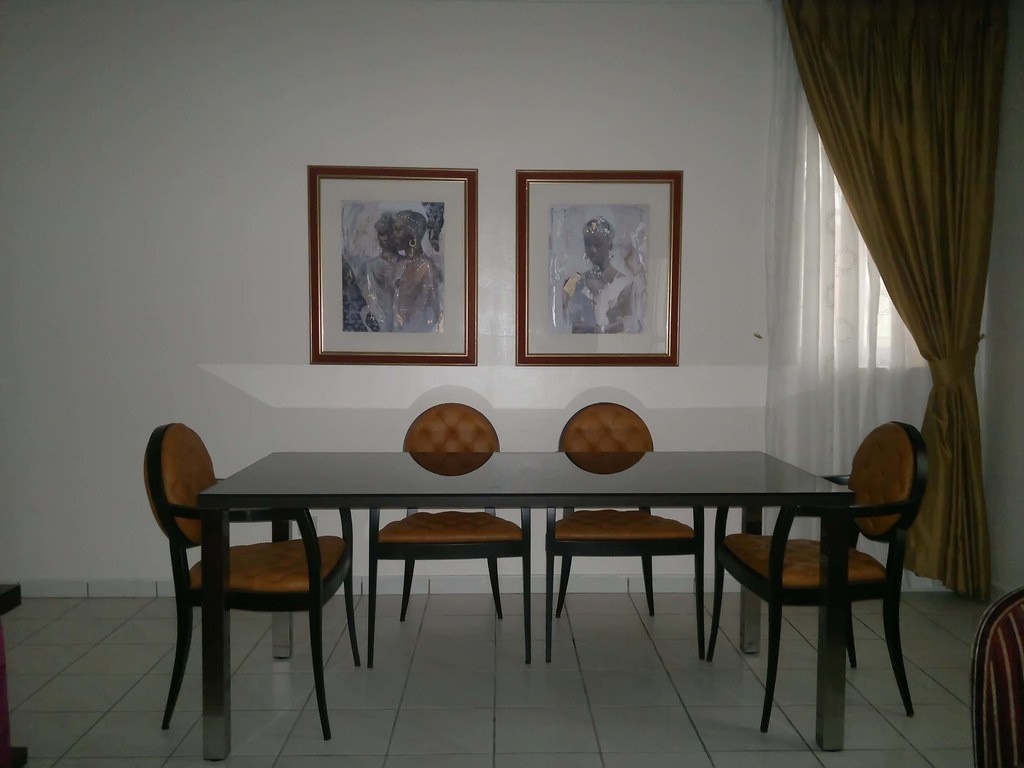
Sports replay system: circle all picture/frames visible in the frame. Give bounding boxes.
[514,169,681,368]
[307,164,481,367]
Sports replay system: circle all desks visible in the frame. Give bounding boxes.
[199,449,858,760]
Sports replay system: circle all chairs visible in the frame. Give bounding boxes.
[367,403,532,670]
[546,401,707,662]
[708,420,930,734]
[142,424,361,741]
